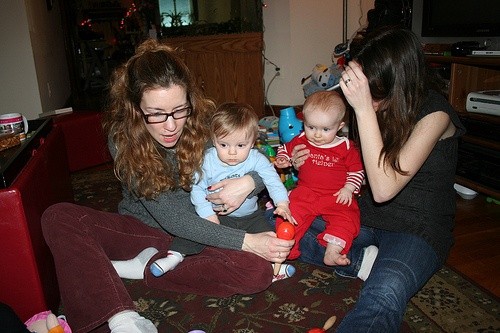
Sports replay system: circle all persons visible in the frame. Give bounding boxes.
[274,91,364,266]
[42,40,292,333]
[291,24,464,333]
[150,101,295,284]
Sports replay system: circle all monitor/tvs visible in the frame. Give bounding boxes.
[412,0,500,54]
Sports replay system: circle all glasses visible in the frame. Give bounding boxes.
[137,96,192,124]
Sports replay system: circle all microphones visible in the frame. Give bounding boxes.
[273,223,295,277]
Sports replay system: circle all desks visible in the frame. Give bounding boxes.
[0,125,73,321]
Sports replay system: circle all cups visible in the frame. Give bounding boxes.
[0,113,28,135]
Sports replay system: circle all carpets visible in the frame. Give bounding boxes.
[71,172,499,333]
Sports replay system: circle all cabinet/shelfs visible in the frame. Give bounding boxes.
[424,53,500,203]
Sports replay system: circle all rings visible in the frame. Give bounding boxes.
[277,251,282,259]
[293,159,298,165]
[345,79,351,84]
